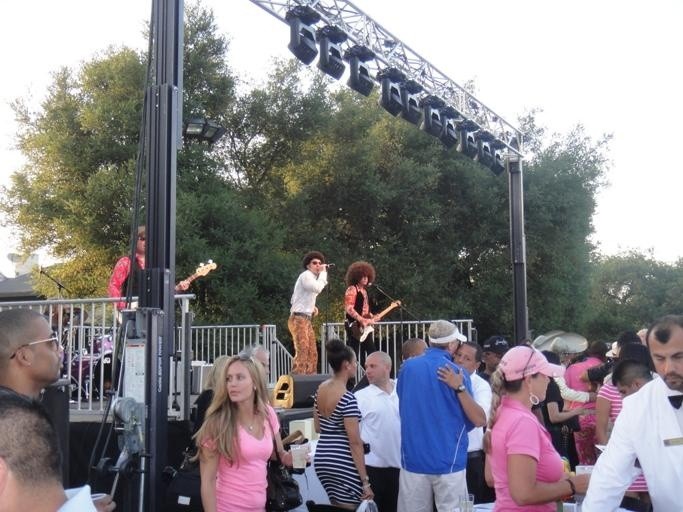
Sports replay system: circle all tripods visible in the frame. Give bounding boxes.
[61,330,89,396]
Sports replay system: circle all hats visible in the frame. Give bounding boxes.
[499,345,567,383]
[483,336,509,354]
[428,327,467,344]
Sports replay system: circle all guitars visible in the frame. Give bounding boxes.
[119,259,217,327]
[350,300,401,341]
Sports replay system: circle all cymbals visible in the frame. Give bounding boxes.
[52,307,87,327]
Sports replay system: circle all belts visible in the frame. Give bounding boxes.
[294,312,312,321]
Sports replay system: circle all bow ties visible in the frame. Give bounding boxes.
[668,395,682,409]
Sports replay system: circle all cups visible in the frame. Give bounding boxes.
[576,465,592,511]
[461,494,475,512]
[290,445,308,469]
[91,493,107,504]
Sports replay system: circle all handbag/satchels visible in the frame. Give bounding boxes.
[264,403,303,512]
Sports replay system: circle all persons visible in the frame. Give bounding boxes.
[104,225,191,315]
[0,308,115,512]
[191,314,683,511]
[0,393,98,512]
[339,258,381,367]
[285,251,329,374]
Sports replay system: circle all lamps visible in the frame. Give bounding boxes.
[284,5,508,177]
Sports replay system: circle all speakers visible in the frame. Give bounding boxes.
[271,374,332,409]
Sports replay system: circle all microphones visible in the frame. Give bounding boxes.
[367,282,381,288]
[326,263,336,269]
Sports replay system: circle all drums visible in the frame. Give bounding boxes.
[88,334,114,354]
[71,353,120,400]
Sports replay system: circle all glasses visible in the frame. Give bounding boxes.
[10,331,59,359]
[518,343,535,380]
[234,352,253,364]
